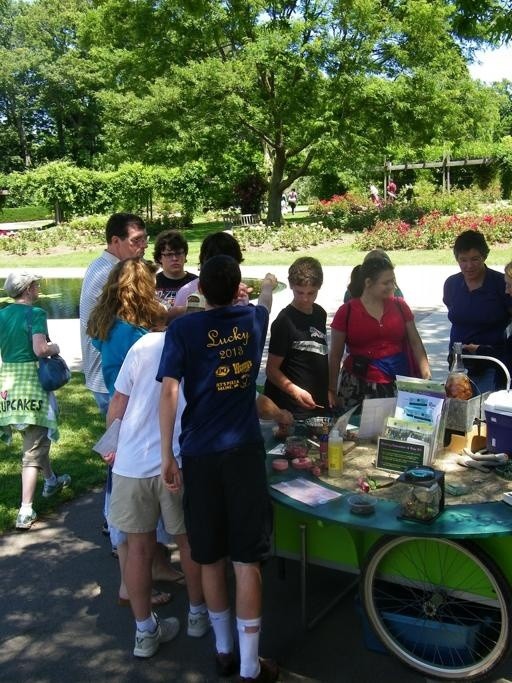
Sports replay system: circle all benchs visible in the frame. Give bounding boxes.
[225,213,261,230]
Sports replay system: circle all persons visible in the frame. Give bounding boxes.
[101,290,207,660]
[0,270,71,530]
[287,188,298,215]
[462,261,512,391]
[172,233,252,306]
[343,250,403,303]
[330,258,431,417]
[444,231,504,396]
[265,257,333,421]
[156,256,276,682]
[370,185,382,210]
[80,213,152,562]
[387,179,397,201]
[86,259,186,608]
[151,231,198,313]
[281,197,287,216]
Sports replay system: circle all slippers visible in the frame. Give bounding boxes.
[155,575,187,587]
[118,591,172,608]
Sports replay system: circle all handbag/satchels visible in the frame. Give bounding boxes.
[28,305,71,392]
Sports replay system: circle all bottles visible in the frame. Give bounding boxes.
[327,429,344,478]
[445,342,473,399]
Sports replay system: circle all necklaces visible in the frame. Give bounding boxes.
[365,300,382,317]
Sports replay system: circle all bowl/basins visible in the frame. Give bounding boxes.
[347,495,377,516]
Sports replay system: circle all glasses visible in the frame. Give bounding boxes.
[161,251,185,258]
[459,255,487,264]
[31,283,41,287]
[132,235,150,244]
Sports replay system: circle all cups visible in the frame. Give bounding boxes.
[280,436,309,457]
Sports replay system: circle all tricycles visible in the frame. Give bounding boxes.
[264,415,512,682]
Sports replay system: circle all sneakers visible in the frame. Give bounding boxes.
[15,509,38,530]
[188,611,212,636]
[239,656,282,683]
[214,643,237,675]
[134,617,179,658]
[43,473,74,497]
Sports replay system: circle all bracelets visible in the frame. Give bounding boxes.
[283,382,292,392]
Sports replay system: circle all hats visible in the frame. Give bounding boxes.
[4,271,41,296]
[174,279,207,308]
